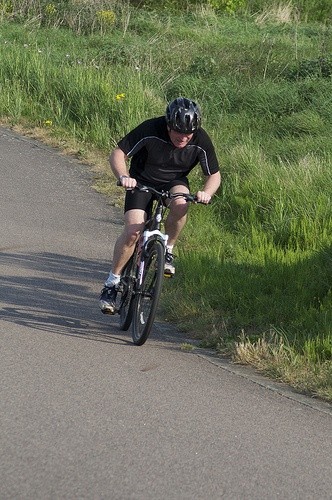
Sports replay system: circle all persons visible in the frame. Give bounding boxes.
[97,97,221,312]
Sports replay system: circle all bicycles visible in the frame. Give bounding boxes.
[102,180,212,346]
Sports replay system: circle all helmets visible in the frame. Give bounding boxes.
[166,97,202,133]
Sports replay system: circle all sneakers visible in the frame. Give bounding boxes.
[164,249,177,277]
[98,284,119,314]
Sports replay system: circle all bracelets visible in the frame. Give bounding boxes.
[120,175,128,179]
